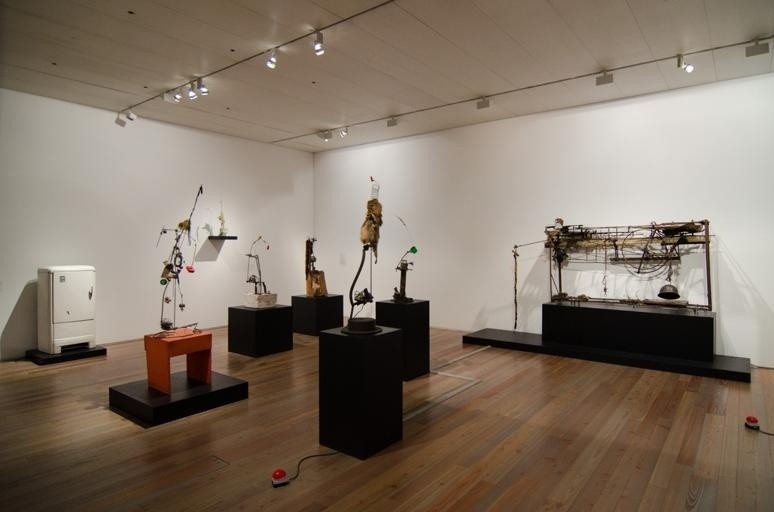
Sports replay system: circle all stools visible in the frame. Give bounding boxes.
[144,328,212,394]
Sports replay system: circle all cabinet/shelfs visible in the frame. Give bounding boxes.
[376,299,429,382]
[463,302,751,383]
[228,307,293,355]
[38,265,96,354]
[320,328,404,457]
[292,295,343,336]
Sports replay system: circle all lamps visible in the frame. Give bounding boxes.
[196,79,209,96]
[314,30,324,56]
[676,53,694,73]
[174,89,186,103]
[267,48,278,70]
[188,84,199,100]
